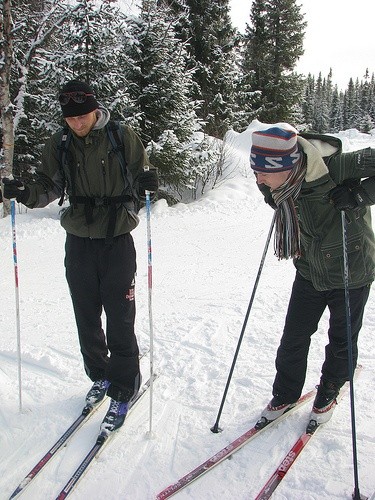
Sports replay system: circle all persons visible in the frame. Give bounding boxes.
[3,80,159,431]
[248,127,375,424]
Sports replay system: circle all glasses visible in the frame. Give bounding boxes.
[59,92,95,105]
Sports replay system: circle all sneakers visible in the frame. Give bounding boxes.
[86,378,110,405]
[103,398,128,430]
[311,377,339,424]
[262,398,297,420]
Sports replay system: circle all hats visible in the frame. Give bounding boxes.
[59,80,98,118]
[249,127,300,172]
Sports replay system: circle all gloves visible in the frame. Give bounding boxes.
[139,171,159,193]
[266,198,277,210]
[2,177,24,200]
[328,187,357,211]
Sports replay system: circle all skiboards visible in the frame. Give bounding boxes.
[8,350,161,500]
[155,363,362,500]
